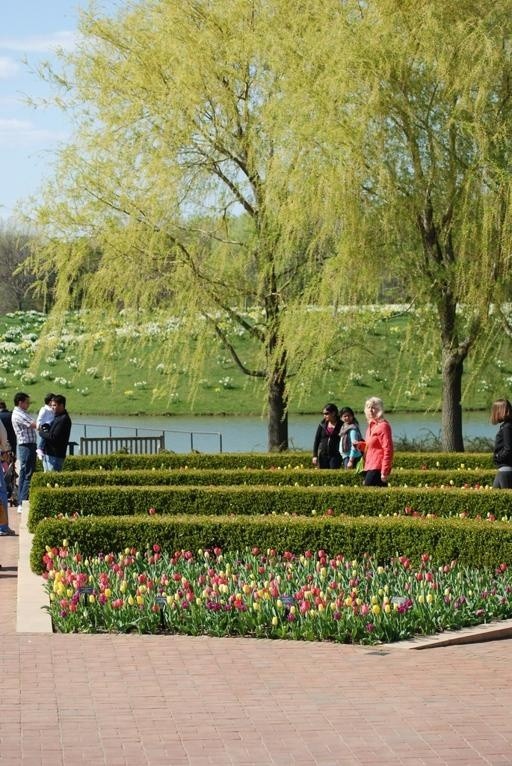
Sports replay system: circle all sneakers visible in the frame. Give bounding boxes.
[17,505,22,514]
[35,448,45,461]
[0,526,16,536]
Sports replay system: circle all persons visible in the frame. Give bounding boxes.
[312,404,345,469]
[491,399,512,488]
[1,420,17,535]
[36,392,56,472]
[34,395,72,472]
[351,396,394,488]
[12,393,40,512]
[0,398,17,467]
[335,406,363,468]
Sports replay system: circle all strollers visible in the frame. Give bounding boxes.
[0,451,20,508]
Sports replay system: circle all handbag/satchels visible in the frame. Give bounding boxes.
[353,454,366,475]
